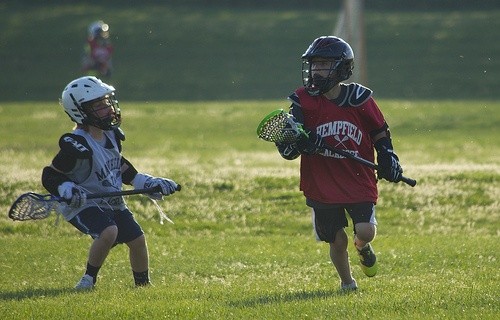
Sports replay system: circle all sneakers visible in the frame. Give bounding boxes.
[75,273,93,290]
[353,234,378,277]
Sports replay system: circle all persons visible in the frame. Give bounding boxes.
[83,21,114,88]
[41,76,181,299]
[278,36,403,291]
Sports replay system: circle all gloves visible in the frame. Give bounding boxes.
[130,173,178,200]
[57,181,86,209]
[281,126,324,162]
[375,137,403,183]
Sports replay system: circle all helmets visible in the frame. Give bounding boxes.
[302,35,355,95]
[60,75,116,124]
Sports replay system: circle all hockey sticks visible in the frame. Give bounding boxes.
[256,109,417,187]
[8,183,181,221]
